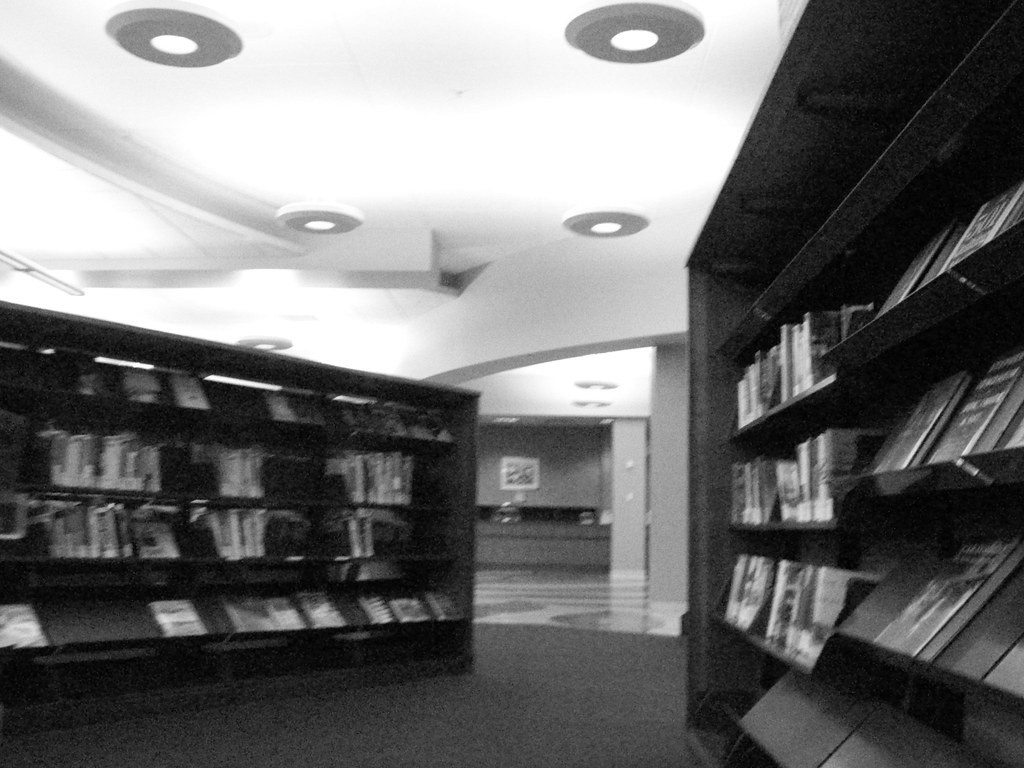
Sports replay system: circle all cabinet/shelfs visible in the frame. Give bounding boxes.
[0,301,482,730]
[684,0,1024,768]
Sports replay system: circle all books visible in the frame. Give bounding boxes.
[1,342,467,648]
[726,183,1024,672]
